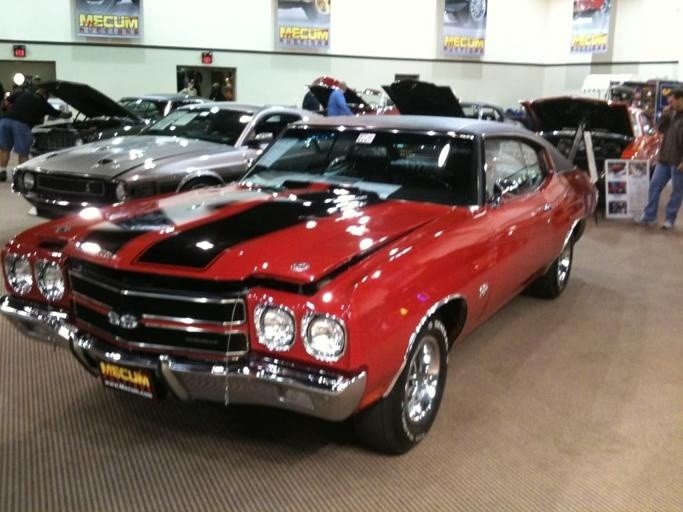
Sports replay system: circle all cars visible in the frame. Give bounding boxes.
[0,115,600,458]
[1,80,209,160]
[609,78,683,132]
[302,72,524,128]
[519,96,659,214]
[10,101,328,223]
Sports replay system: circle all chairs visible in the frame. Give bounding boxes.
[330,143,475,200]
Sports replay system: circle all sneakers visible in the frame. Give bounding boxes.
[0,170,7,182]
[633,214,674,229]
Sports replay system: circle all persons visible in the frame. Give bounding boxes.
[610,83,655,140]
[176,77,200,99]
[207,82,227,101]
[630,90,682,231]
[0,87,72,183]
[301,90,324,113]
[325,82,355,117]
[0,72,42,119]
